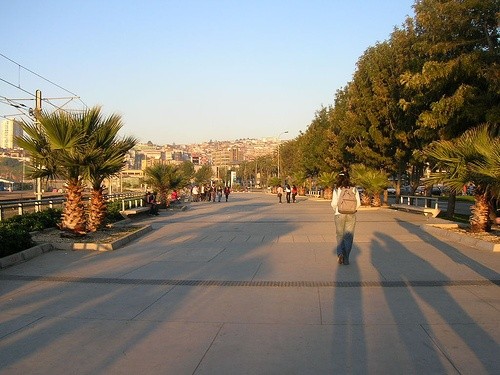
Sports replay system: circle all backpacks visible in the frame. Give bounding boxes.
[339,186,357,213]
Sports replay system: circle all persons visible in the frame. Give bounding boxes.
[151,191,160,215]
[170,182,223,204]
[277,185,283,205]
[224,184,230,202]
[291,185,298,203]
[285,185,291,203]
[331,171,361,265]
[142,192,156,217]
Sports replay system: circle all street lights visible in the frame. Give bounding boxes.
[277,131,289,179]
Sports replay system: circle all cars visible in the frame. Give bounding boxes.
[306,183,480,197]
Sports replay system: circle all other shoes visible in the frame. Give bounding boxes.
[338,256,342,265]
[208,200,228,202]
[151,213,160,216]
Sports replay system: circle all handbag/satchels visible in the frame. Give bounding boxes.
[278,193,282,197]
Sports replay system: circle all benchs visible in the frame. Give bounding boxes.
[305,190,321,198]
[392,195,442,218]
[119,195,151,218]
